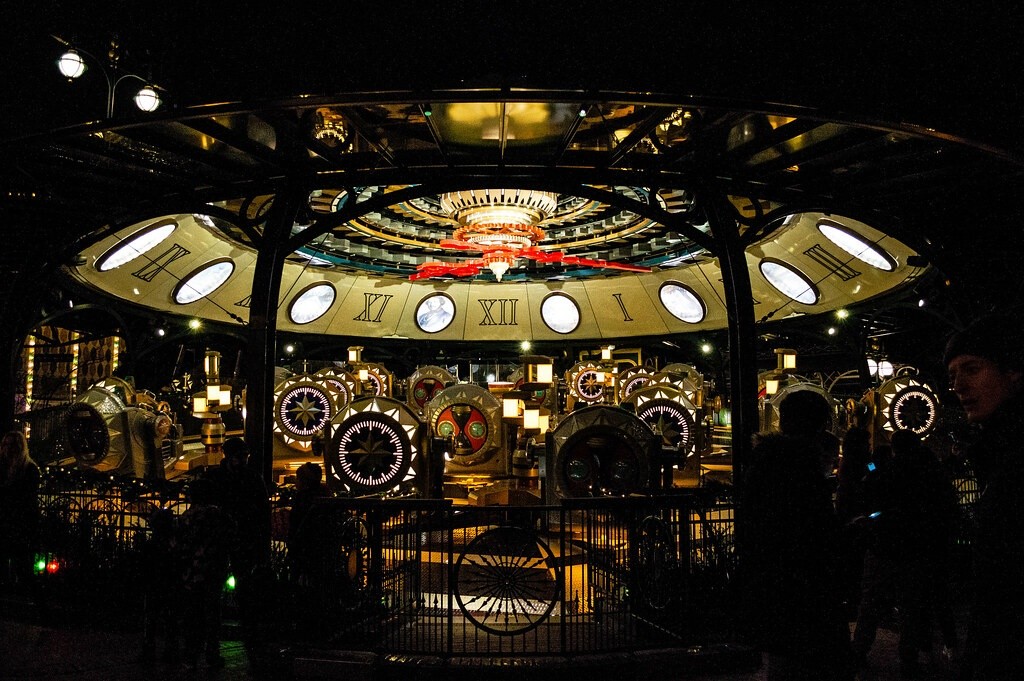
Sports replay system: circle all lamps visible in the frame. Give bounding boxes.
[440,188,558,284]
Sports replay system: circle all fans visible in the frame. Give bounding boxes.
[408,238,653,283]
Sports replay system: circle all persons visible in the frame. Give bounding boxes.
[284,462,334,651]
[735,391,860,681]
[140,524,184,665]
[195,437,270,627]
[837,428,892,668]
[181,505,230,668]
[943,317,1024,681]
[912,452,963,667]
[0,431,42,601]
[815,430,865,681]
[877,430,940,681]
